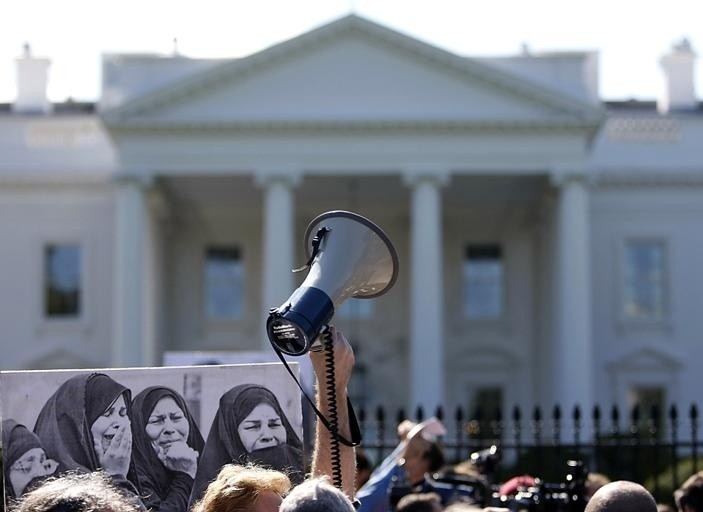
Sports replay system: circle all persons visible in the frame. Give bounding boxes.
[26,372,133,482]
[120,385,205,511]
[186,381,302,510]
[279,326,356,512]
[13,473,134,512]
[355,420,702,512]
[192,465,291,512]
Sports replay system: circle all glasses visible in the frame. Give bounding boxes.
[8,459,59,476]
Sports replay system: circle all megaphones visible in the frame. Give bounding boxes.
[265,209,400,356]
